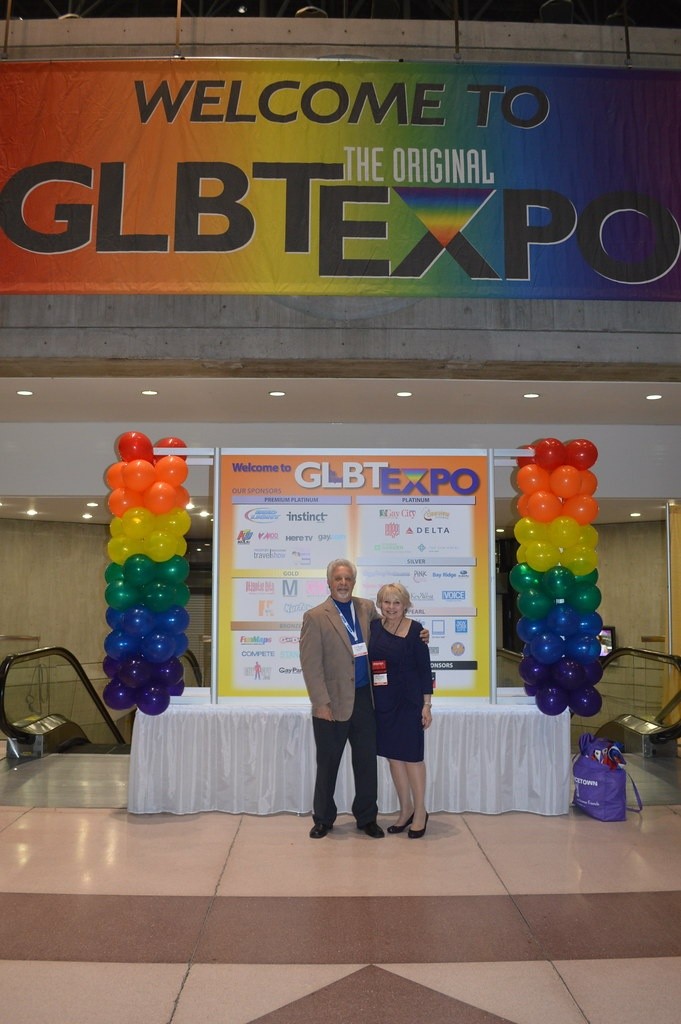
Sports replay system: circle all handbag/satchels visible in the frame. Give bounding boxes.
[572,733,642,821]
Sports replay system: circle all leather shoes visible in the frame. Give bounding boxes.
[356,820,384,838]
[310,818,333,838]
[387,812,429,838]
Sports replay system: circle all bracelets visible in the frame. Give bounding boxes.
[424,702,432,707]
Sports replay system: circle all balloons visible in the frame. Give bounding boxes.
[101,432,191,716]
[510,438,603,718]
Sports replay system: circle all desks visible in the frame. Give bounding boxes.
[128,687,571,817]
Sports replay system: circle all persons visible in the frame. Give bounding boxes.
[299,559,430,839]
[367,583,433,838]
